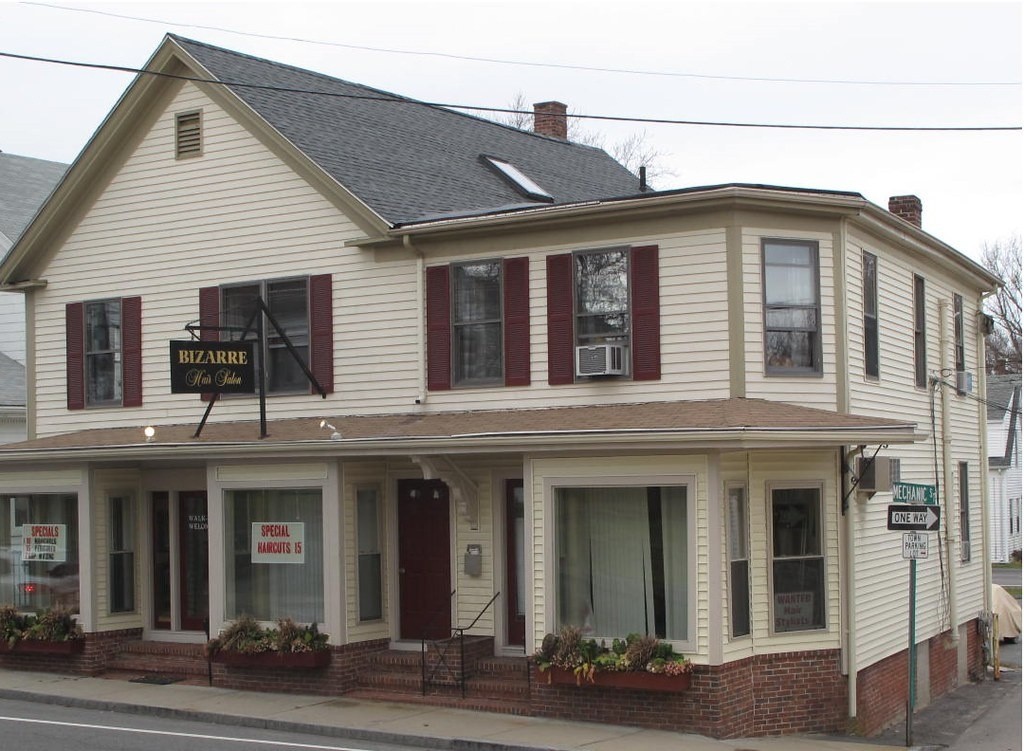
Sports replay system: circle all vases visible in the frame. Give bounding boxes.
[210,650,329,669]
[539,665,691,692]
[0,639,81,655]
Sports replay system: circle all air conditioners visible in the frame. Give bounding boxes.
[958,372,973,394]
[857,455,901,493]
[575,343,625,380]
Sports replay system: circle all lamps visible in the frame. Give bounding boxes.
[144,427,158,442]
[321,420,344,441]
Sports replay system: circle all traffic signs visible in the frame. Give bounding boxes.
[885,502,943,532]
[901,532,929,560]
[891,483,938,506]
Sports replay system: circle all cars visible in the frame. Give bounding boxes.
[13,558,79,598]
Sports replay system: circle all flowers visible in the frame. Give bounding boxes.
[205,616,330,658]
[0,604,86,648]
[536,629,694,688]
